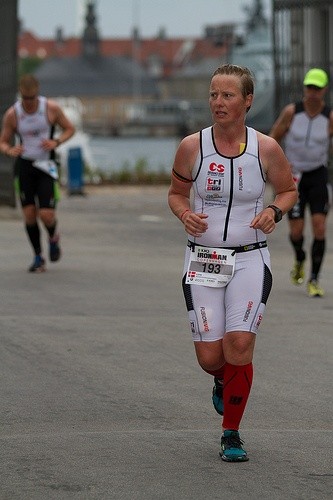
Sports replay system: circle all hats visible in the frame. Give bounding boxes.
[304,68,328,88]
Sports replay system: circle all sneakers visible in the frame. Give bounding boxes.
[50,234,60,262]
[291,258,305,285]
[212,386,224,415]
[219,430,249,463]
[29,259,45,274]
[307,282,325,297]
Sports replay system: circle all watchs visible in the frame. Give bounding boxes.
[266,205,282,223]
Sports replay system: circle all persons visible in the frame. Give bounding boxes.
[0,75,76,274]
[167,64,299,464]
[267,67,333,299]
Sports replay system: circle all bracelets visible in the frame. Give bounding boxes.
[53,139,60,147]
[180,209,192,222]
